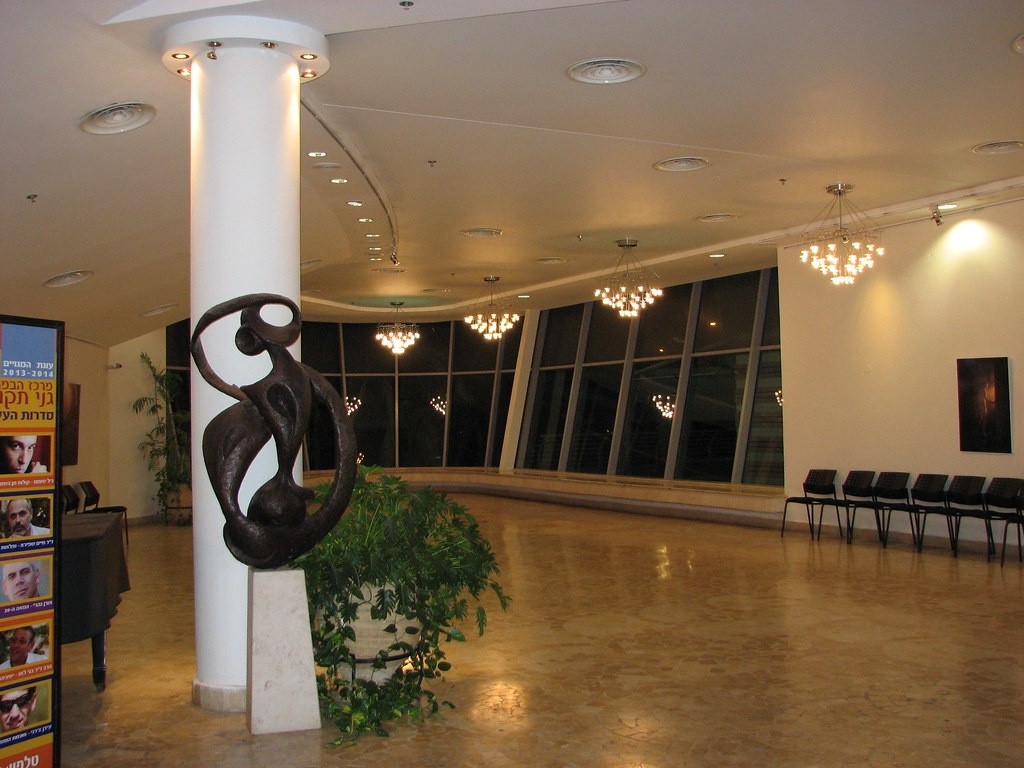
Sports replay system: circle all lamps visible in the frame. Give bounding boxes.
[804,184,886,286]
[653,384,673,419]
[930,208,944,226]
[590,239,664,317]
[773,373,783,406]
[431,391,447,415]
[462,276,520,341]
[345,369,362,415]
[375,302,420,355]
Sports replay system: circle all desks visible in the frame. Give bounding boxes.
[60,512,132,680]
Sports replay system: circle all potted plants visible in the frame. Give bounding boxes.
[304,465,513,750]
[133,354,193,525]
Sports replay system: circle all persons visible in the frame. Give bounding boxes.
[0,626,48,670]
[0,686,37,732]
[0,436,47,474]
[8,499,50,538]
[2,560,40,601]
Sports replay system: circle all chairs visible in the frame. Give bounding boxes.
[63,486,79,514]
[79,482,129,546]
[919,475,996,558]
[886,473,952,551]
[818,469,882,543]
[955,477,1024,568]
[846,471,916,547]
[776,468,843,540]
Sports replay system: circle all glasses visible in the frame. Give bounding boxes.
[0,691,36,713]
